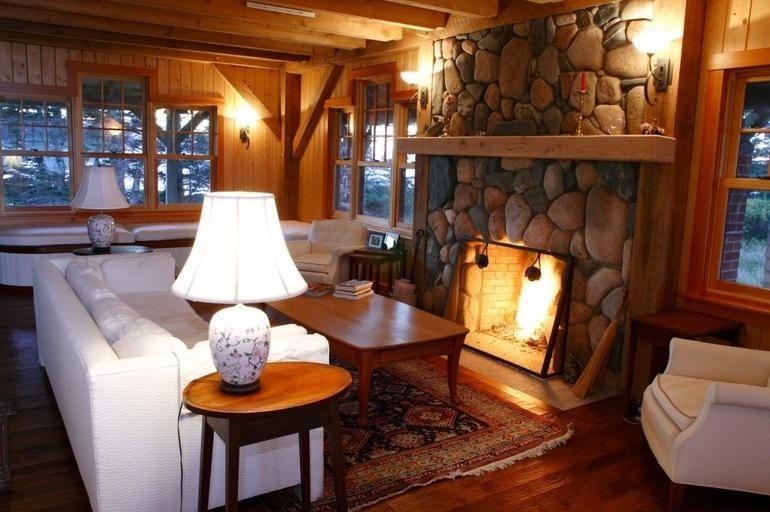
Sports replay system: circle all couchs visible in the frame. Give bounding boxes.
[29,254,334,512]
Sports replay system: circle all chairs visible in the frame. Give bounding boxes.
[282,216,365,291]
[639,341,770,510]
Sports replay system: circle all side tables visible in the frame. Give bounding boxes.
[180,363,356,512]
[624,309,746,418]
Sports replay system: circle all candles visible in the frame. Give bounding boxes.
[581,72,586,91]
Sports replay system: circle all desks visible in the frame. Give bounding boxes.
[347,247,408,297]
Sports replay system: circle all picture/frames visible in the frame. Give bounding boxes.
[367,231,385,250]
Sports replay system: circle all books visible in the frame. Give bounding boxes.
[335,287,371,296]
[332,290,375,300]
[334,278,373,292]
[302,281,334,299]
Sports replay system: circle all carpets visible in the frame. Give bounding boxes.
[278,318,574,512]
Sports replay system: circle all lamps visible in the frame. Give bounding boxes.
[173,186,311,394]
[71,166,131,252]
